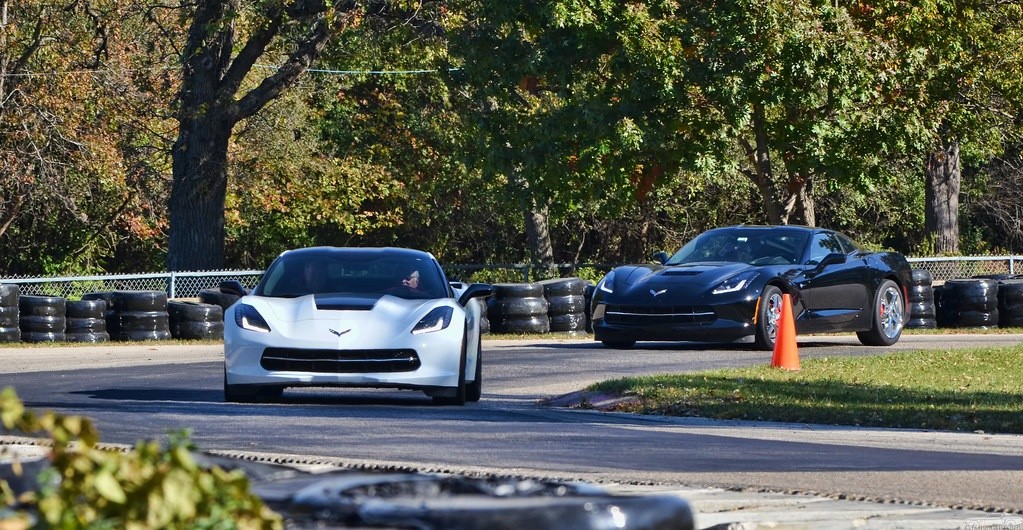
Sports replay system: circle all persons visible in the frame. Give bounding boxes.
[397,265,420,289]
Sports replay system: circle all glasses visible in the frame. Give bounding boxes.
[400,275,415,281]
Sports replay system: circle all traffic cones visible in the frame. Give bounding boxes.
[771,294,800,370]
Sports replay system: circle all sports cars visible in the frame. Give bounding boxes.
[219,246,493,406]
[589,225,914,350]
